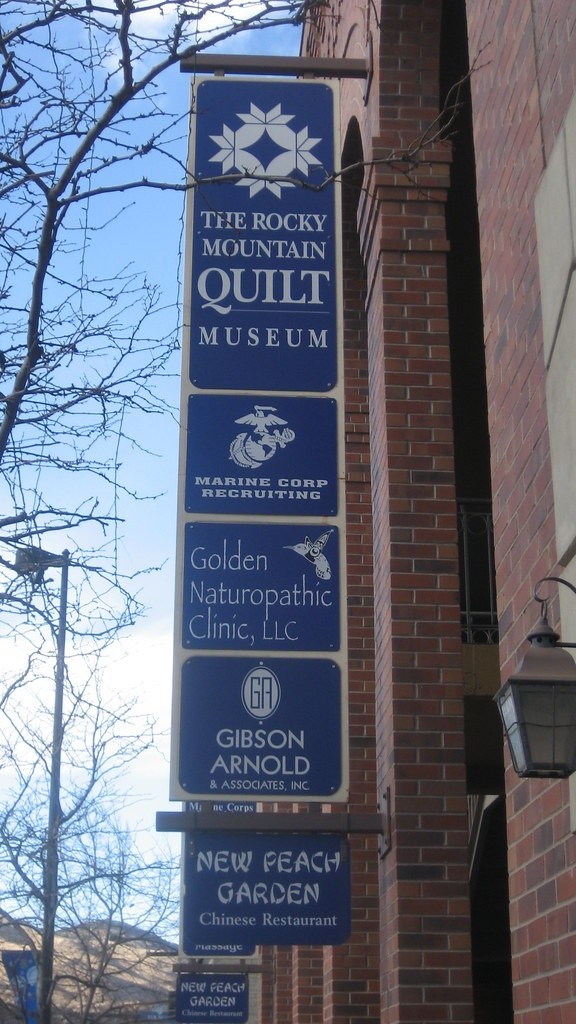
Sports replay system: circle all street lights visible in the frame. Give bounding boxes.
[14,545,75,1024]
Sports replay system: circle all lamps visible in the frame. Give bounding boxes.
[492,577,576,780]
